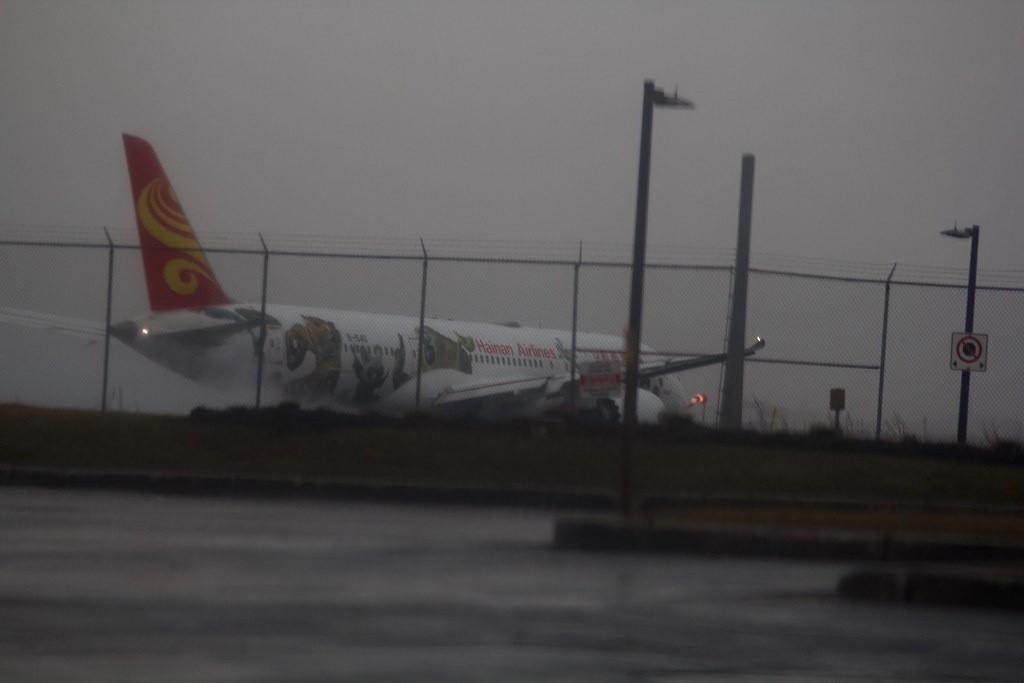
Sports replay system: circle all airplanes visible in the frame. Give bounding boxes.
[1,133,766,427]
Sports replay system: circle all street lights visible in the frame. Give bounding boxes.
[617,80,697,511]
[940,223,982,439]
